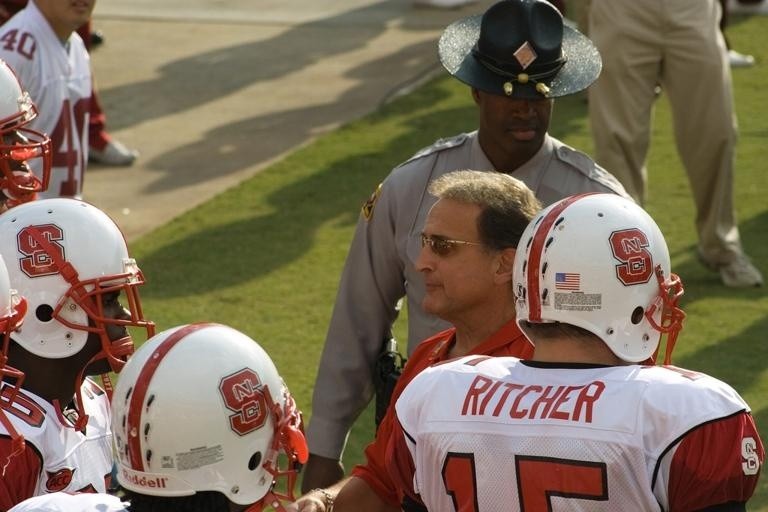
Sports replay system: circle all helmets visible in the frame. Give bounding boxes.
[0,59,50,214]
[1,198,154,411]
[111,323,309,512]
[511,190,686,365]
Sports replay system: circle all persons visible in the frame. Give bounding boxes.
[0,0,140,209]
[302,1,638,495]
[384,193,766,510]
[585,1,765,291]
[282,167,546,512]
[718,2,755,68]
[0,191,148,512]
[11,322,309,512]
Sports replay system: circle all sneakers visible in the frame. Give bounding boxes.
[727,49,757,68]
[89,137,138,166]
[694,244,766,290]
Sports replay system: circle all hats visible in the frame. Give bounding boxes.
[438,0,603,100]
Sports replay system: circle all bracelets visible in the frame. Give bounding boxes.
[312,482,334,511]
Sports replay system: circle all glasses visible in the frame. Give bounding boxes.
[421,234,490,254]
